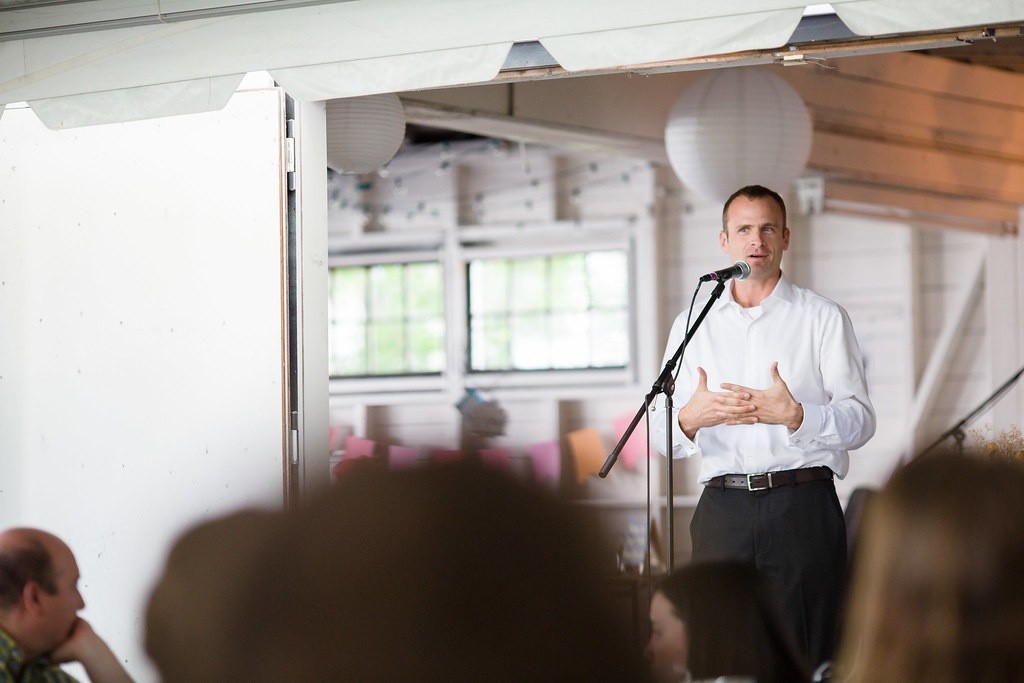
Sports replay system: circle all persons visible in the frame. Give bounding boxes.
[147,437,1024,683]
[760,182,760,185]
[0,527,136,683]
[644,183,876,683]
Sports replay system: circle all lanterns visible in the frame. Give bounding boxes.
[323,90,404,174]
[662,67,816,199]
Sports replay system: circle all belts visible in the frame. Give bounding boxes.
[704,466,833,491]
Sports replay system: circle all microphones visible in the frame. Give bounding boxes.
[700,260,750,281]
